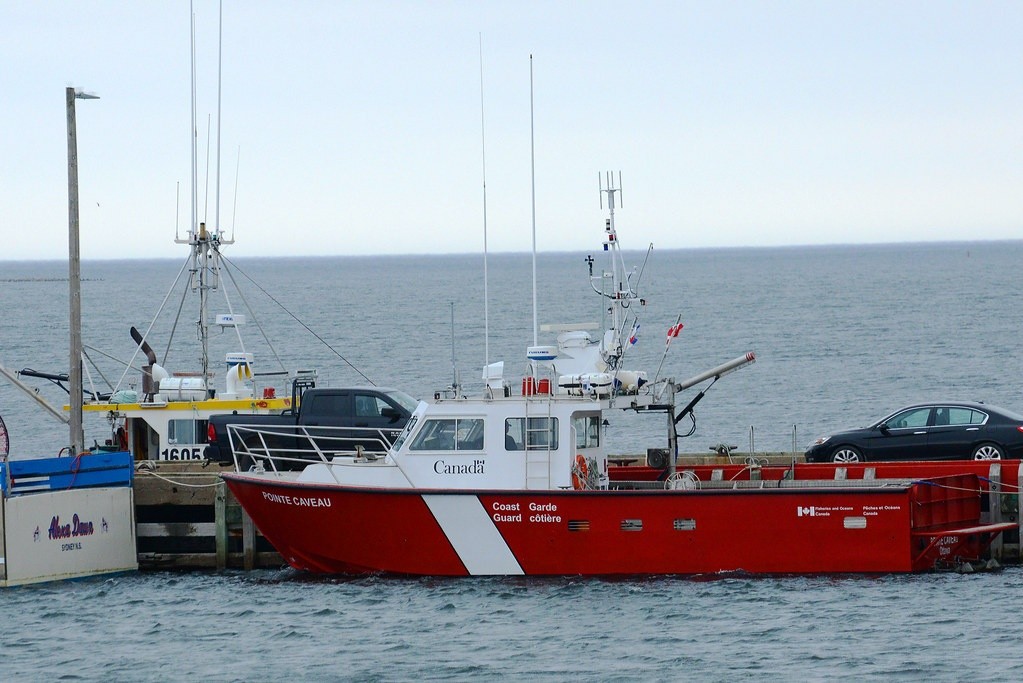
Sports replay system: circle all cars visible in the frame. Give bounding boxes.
[800,400,1023,462]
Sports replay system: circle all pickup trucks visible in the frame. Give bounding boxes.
[199,376,483,471]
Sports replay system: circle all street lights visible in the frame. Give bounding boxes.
[64,83,106,451]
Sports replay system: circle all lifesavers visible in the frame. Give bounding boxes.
[571,454,588,492]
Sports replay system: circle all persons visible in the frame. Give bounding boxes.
[505,421,517,450]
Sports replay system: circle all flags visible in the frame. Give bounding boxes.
[667,323,684,344]
[629,325,640,348]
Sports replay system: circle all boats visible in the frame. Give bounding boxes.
[62,325,301,461]
[222,160,1023,579]
